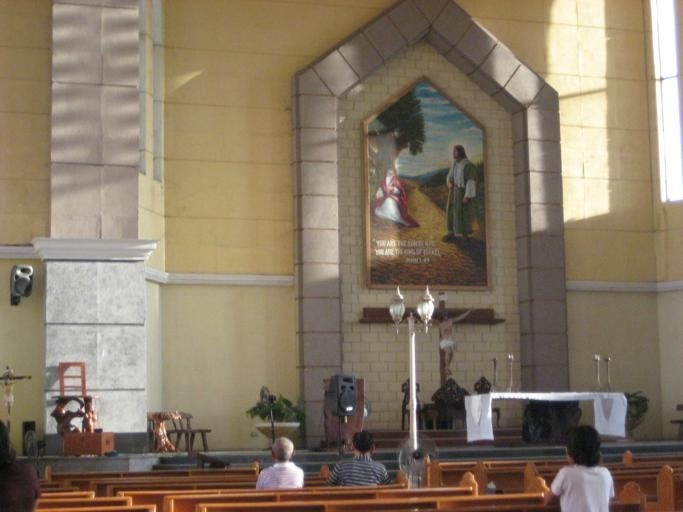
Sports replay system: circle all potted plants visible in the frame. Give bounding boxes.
[246,385,305,450]
[616,391,649,443]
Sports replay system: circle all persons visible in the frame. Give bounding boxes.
[256,437,304,491]
[374,168,419,227]
[320,430,393,486]
[441,145,478,250]
[0,420,41,512]
[543,426,614,512]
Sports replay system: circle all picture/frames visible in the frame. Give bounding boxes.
[363,74,491,291]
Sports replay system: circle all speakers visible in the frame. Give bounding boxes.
[326,375,357,416]
[11,264,33,297]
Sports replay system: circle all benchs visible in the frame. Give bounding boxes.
[37,451,683,512]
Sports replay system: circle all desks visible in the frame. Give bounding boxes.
[148,411,182,450]
[465,392,628,446]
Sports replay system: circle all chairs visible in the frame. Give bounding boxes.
[166,421,190,452]
[51,361,97,435]
[172,414,211,451]
